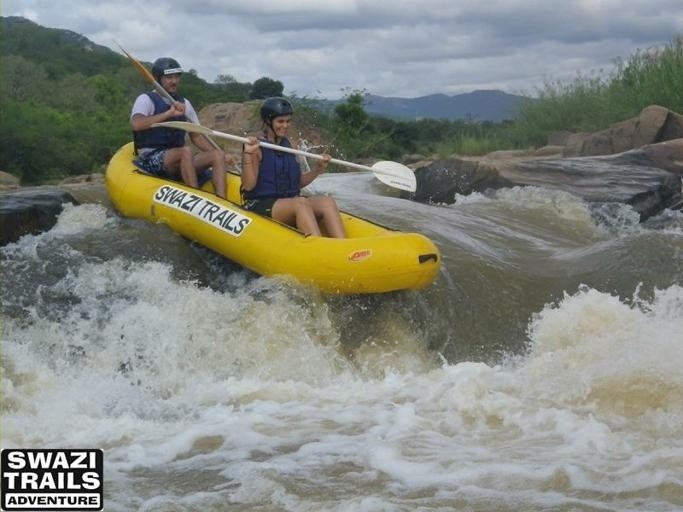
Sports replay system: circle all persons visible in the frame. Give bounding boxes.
[237,96,350,242]
[129,56,230,200]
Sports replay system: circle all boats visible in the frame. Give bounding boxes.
[106,142,440,294]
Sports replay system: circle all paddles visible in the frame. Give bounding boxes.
[151,121,417,192]
[112,39,241,174]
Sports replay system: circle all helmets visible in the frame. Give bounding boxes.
[260,98,293,128]
[153,58,183,84]
